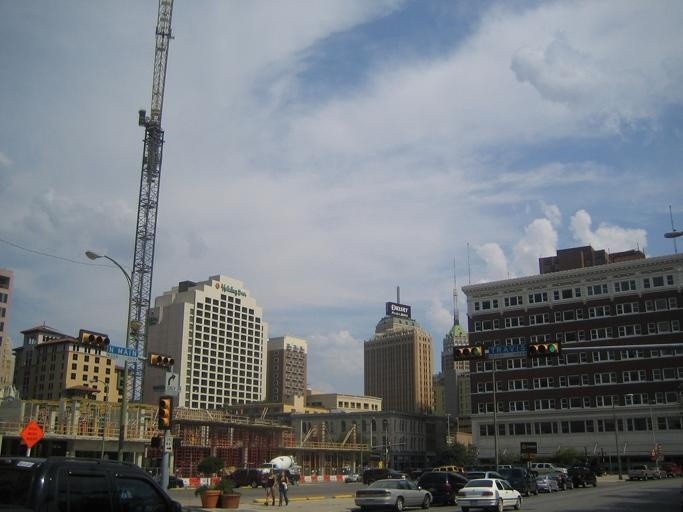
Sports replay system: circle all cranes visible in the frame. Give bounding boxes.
[120,0,178,399]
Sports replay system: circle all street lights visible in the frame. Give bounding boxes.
[609,391,635,480]
[80,249,133,463]
[88,378,110,461]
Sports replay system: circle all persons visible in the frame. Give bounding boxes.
[274,470,290,506]
[264,469,278,506]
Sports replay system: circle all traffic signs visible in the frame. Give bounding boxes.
[487,343,527,360]
[107,344,138,358]
[163,435,172,452]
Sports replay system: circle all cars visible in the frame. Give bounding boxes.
[417,464,597,506]
[352,477,433,511]
[224,468,271,489]
[453,477,523,512]
[343,474,361,481]
[153,474,184,488]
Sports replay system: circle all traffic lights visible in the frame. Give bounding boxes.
[451,344,484,362]
[525,341,560,358]
[157,395,173,430]
[77,328,110,353]
[147,351,175,371]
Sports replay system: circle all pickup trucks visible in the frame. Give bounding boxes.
[626,462,682,482]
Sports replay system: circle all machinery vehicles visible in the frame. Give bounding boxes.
[260,454,301,486]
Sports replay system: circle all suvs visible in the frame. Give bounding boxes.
[0,455,182,511]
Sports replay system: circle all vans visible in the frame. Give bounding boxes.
[362,467,407,486]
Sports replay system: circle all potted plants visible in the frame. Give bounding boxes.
[217,478,241,508]
[195,486,221,508]
